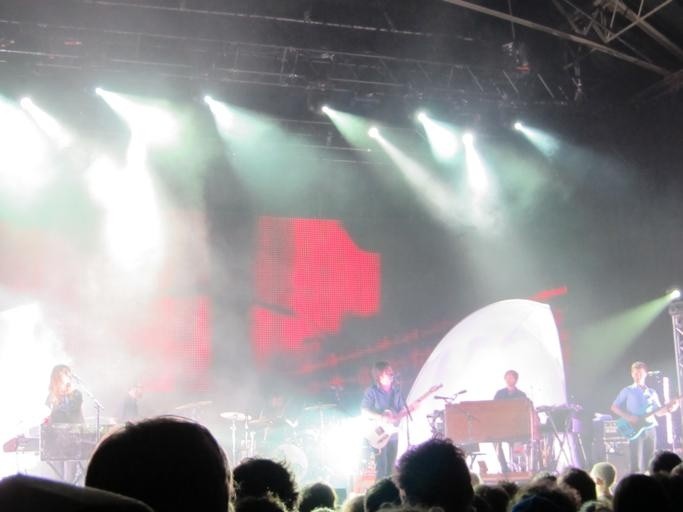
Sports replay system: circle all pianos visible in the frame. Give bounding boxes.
[4,422,108,453]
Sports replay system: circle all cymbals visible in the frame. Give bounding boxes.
[176,400,212,408]
[221,411,245,421]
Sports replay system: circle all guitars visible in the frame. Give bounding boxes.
[361,384,442,449]
[615,394,683,440]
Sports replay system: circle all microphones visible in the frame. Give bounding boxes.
[65,371,74,380]
[458,389,467,394]
[434,395,451,400]
[391,375,401,386]
[648,371,660,377]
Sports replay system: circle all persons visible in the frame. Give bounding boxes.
[122,385,143,418]
[260,391,296,451]
[494,369,528,472]
[360,360,421,480]
[43,363,84,481]
[611,359,678,473]
[84,417,682,512]
